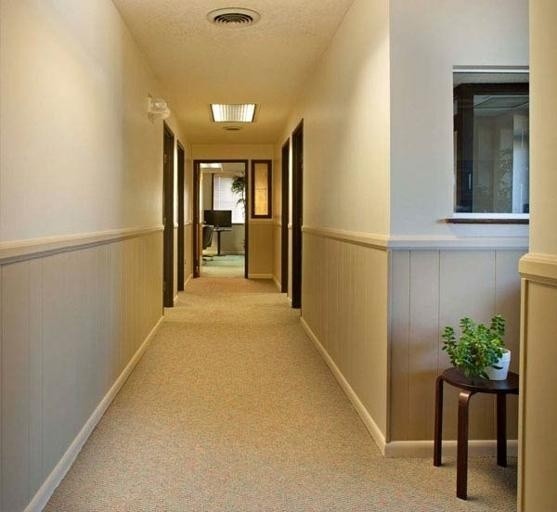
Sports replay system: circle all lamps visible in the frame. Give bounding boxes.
[146,96,171,123]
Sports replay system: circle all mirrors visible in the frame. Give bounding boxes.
[250,156,272,218]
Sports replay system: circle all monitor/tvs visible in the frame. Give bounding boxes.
[204,210,231,228]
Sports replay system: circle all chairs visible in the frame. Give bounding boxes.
[202,224,214,266]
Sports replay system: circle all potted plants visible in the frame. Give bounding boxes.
[440,313,511,381]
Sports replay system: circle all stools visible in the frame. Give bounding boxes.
[430,364,518,500]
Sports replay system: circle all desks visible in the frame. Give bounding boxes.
[210,227,232,258]
